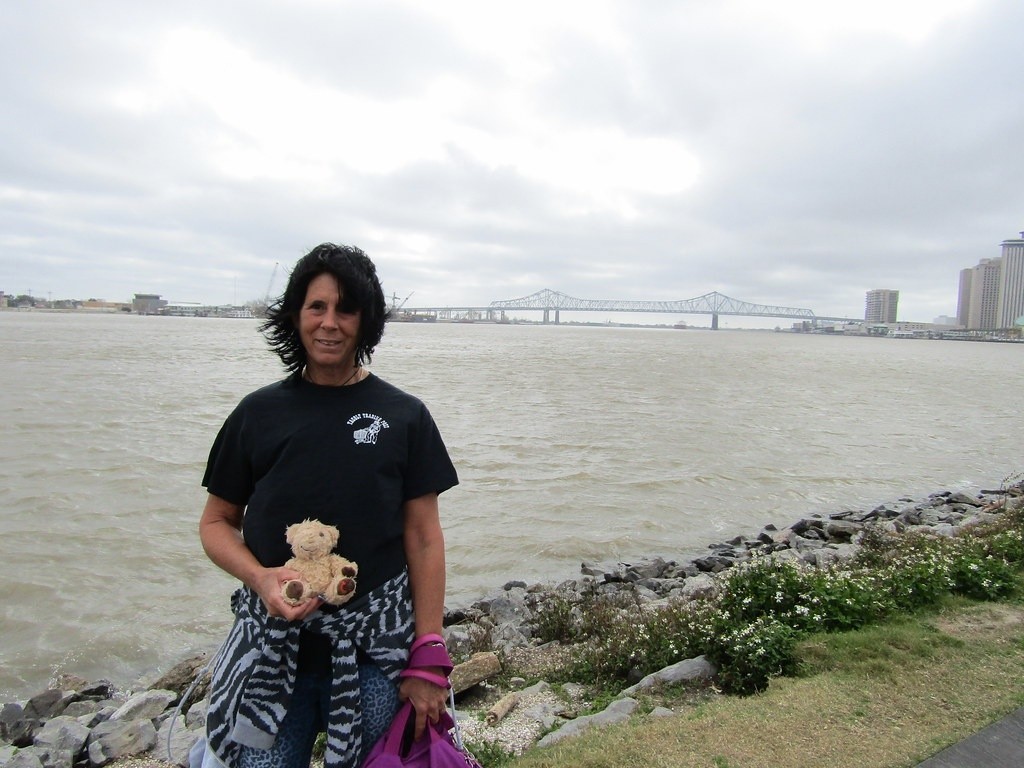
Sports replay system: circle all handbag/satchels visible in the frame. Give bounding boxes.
[362,634,482,768]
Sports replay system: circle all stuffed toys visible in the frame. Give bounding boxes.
[279,519,358,608]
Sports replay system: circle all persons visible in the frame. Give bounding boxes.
[197,243,456,768]
